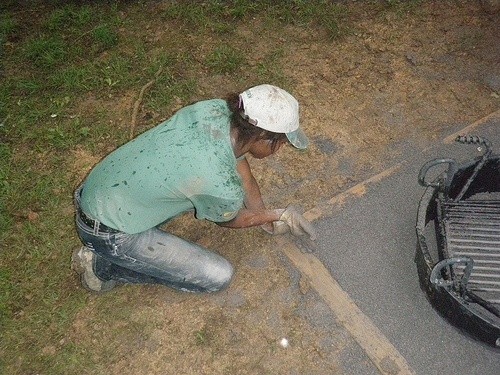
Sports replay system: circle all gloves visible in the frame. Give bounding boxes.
[280,203,315,252]
[264,220,289,235]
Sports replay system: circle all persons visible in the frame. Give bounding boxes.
[69,84,317,296]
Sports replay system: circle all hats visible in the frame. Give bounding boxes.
[239,84,310,150]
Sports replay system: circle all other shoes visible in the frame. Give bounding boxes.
[72,244,104,293]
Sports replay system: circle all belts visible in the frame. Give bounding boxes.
[77,206,119,233]
